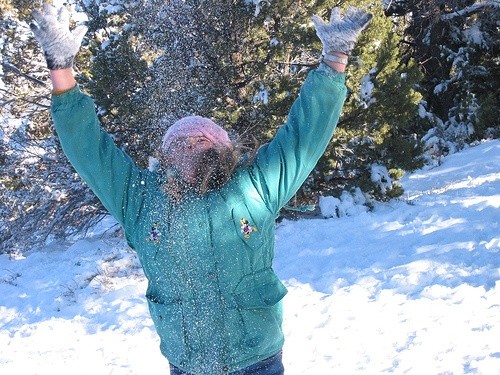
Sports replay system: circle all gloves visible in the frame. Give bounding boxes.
[311,7,373,57]
[28,3,88,68]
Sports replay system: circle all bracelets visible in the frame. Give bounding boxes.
[323,54,348,65]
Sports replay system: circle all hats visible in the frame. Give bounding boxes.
[161,117,230,151]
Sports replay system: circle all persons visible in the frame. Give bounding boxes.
[25,5,374,375]
